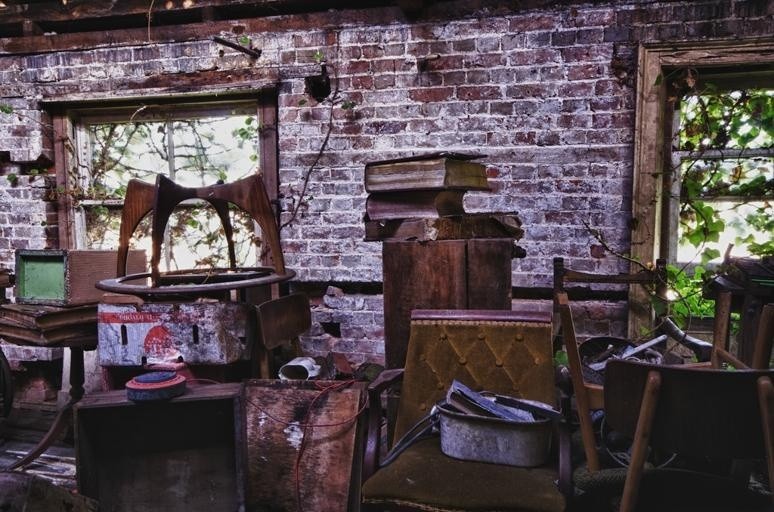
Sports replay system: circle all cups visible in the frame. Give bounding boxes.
[277,356,322,381]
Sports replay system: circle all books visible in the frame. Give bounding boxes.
[362,152,525,243]
